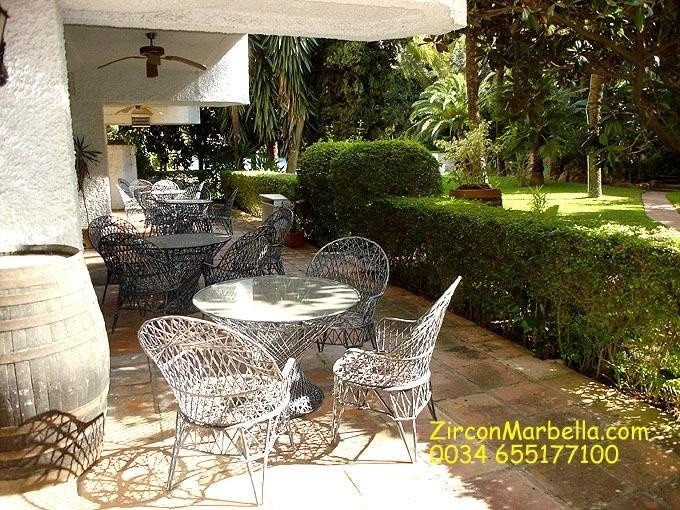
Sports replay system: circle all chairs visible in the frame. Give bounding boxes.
[87,178,296,334]
[137,315,297,507]
[331,276,462,463]
[300,236,389,365]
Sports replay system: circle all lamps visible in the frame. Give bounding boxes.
[1,6,11,88]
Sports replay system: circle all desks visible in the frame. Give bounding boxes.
[192,274,361,420]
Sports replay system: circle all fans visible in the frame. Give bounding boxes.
[115,105,154,118]
[96,33,207,79]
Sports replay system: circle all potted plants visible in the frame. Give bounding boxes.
[71,134,105,252]
[442,118,505,208]
